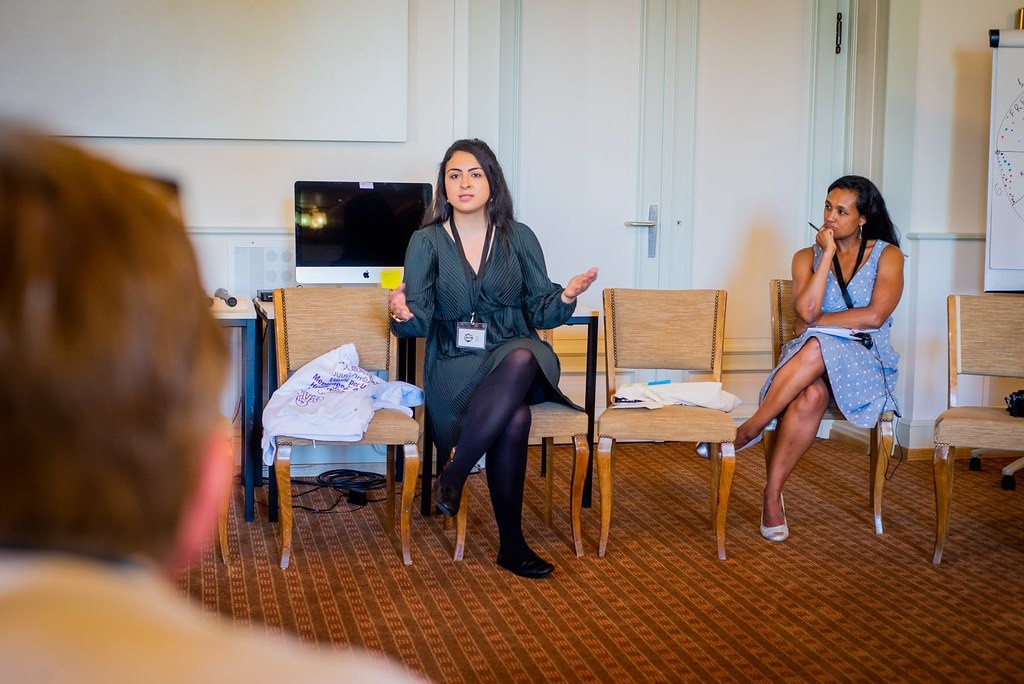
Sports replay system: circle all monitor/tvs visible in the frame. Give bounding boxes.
[296,181,434,283]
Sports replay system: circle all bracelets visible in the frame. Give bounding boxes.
[390,312,404,323]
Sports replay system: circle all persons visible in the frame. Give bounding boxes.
[696,175,909,542]
[0,113,432,683]
[387,139,599,577]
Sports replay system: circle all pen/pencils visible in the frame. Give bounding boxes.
[647,380,671,385]
[808,222,819,231]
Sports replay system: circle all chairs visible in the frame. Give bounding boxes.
[442,327,591,563]
[271,287,421,570]
[596,287,737,559]
[932,294,1024,566]
[762,278,894,534]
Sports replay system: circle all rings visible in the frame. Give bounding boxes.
[793,332,796,336]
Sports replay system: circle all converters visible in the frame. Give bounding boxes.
[348,488,368,505]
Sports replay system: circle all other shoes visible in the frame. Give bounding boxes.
[695,429,763,459]
[760,495,789,541]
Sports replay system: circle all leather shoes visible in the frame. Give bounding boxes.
[434,473,458,517]
[496,550,555,577]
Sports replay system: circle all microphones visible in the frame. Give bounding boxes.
[214,288,238,307]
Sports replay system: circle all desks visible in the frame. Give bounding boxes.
[202,293,276,525]
